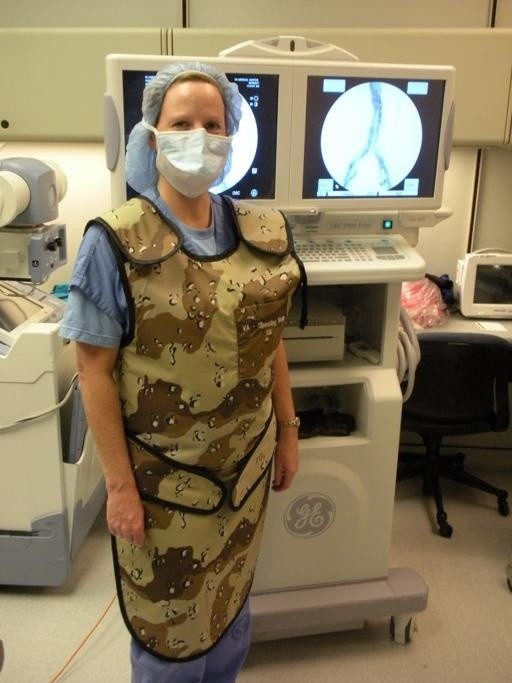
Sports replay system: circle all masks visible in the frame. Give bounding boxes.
[149,124,233,201]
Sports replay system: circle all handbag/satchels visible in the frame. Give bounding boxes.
[426,273,460,315]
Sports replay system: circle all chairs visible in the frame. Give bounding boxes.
[395,330,512,538]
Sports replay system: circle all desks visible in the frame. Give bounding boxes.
[417,309,512,348]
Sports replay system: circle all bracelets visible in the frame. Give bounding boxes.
[273,415,301,430]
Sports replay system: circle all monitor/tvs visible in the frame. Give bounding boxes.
[287,62,456,209]
[455,253,512,319]
[103,54,280,214]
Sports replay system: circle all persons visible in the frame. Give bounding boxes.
[54,61,304,683]
